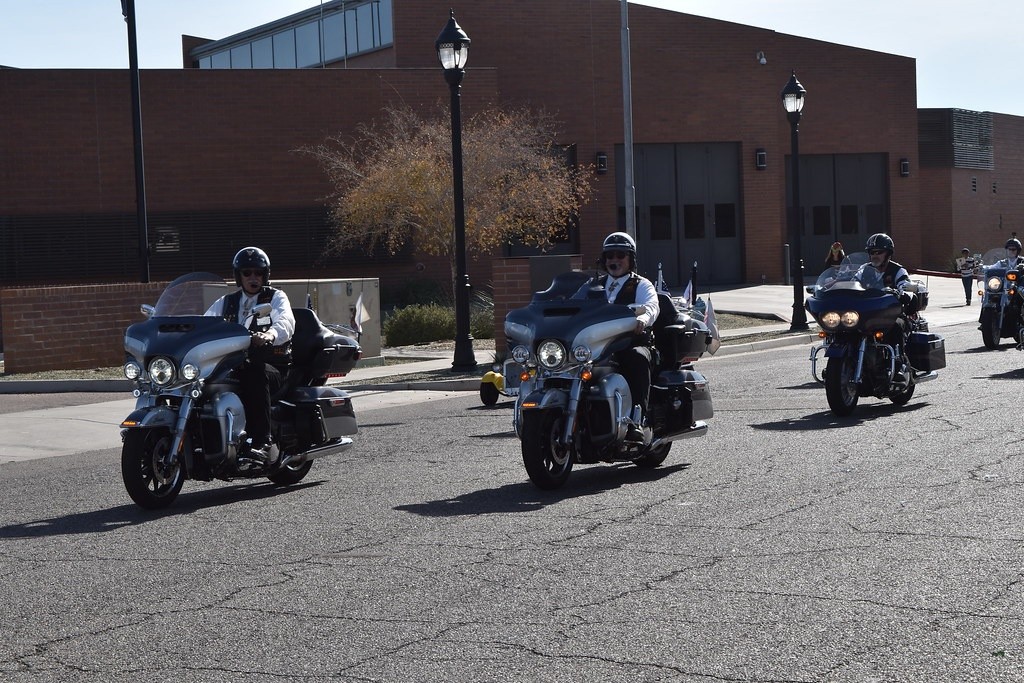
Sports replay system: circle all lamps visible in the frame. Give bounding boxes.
[756,49,767,66]
[756,148,768,169]
[900,158,909,176]
[596,152,609,175]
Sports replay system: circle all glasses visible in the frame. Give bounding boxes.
[1008,247,1017,251]
[605,251,627,260]
[869,250,880,255]
[239,269,263,277]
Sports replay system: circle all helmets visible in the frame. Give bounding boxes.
[1005,238,1022,254]
[865,233,895,256]
[602,232,637,271]
[233,246,271,287]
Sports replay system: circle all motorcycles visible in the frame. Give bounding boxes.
[119,271,364,510]
[977,247,1024,349]
[503,268,713,491]
[805,251,946,416]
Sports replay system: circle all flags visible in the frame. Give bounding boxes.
[353,295,370,332]
[704,299,721,355]
[304,293,315,312]
[683,277,692,305]
[654,270,670,297]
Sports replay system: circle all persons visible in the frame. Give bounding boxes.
[571,231,660,441]
[825,242,849,277]
[978,238,1024,331]
[204,246,295,460]
[957,248,976,306]
[851,233,913,382]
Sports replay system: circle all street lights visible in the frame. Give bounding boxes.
[435,8,481,374]
[780,68,809,331]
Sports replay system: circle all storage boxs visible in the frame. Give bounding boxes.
[676,320,711,363]
[657,371,714,421]
[326,335,362,373]
[908,332,946,371]
[907,281,928,310]
[293,386,358,444]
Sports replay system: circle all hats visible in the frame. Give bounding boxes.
[961,247,969,252]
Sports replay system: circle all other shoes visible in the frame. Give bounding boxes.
[251,444,269,462]
[894,371,906,384]
[624,422,645,442]
